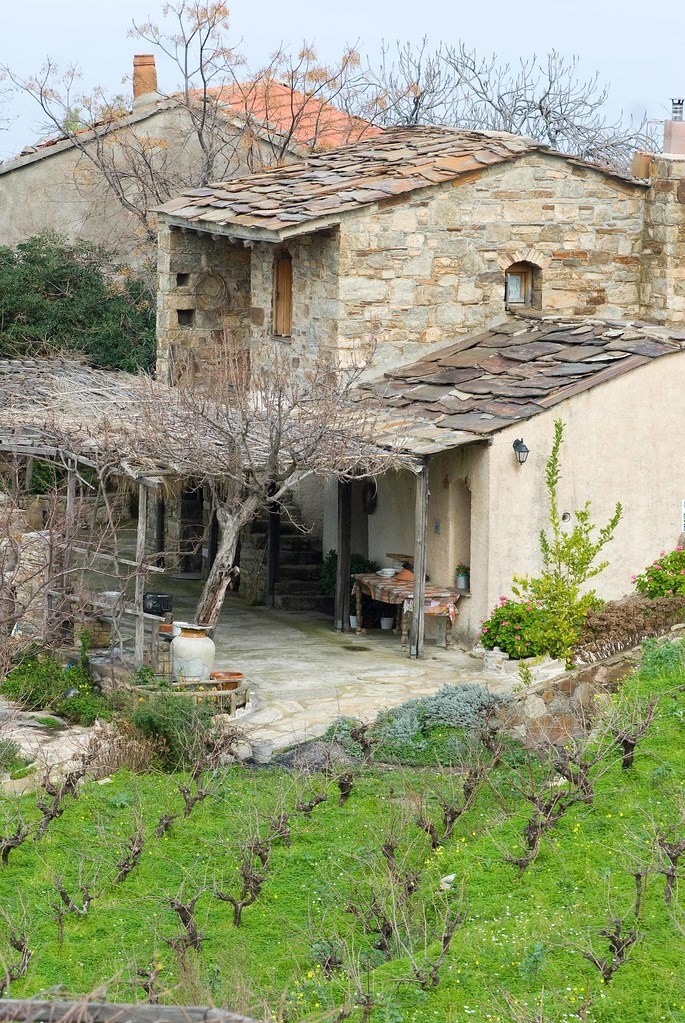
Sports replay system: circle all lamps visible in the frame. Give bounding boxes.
[513,437,530,465]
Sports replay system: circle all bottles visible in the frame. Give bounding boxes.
[170,625,215,682]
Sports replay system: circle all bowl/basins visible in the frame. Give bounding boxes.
[210,671,244,690]
[159,623,172,632]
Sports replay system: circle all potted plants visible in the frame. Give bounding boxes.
[454,560,470,589]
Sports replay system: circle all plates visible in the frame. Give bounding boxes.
[376,568,396,576]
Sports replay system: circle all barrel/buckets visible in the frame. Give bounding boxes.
[143,592,173,613]
[100,591,121,617]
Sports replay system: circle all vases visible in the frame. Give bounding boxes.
[211,672,244,691]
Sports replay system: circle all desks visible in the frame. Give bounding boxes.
[350,571,461,650]
